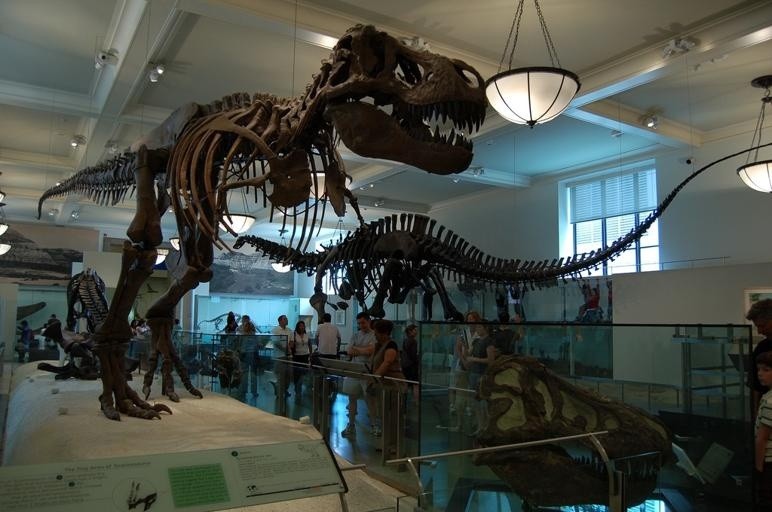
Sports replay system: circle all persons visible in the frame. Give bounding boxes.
[15,319,35,363]
[605,279,612,321]
[135,319,151,358]
[581,279,603,315]
[741,299,772,421]
[50,313,57,319]
[217,312,260,396]
[576,279,593,318]
[495,283,511,324]
[508,282,528,322]
[341,312,420,452]
[271,314,342,430]
[435,311,531,465]
[128,319,138,357]
[173,318,184,340]
[752,351,771,501]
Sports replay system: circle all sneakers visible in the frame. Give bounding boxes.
[251,390,259,397]
[342,423,356,436]
[285,390,292,397]
[370,424,396,454]
[449,403,485,439]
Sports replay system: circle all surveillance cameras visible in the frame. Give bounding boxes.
[686,157,695,164]
[98,51,108,62]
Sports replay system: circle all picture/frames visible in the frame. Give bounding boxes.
[335,310,346,326]
[742,287,772,344]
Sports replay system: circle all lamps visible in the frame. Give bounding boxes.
[48,208,80,220]
[484,0,581,130]
[0,191,11,256]
[94,50,117,70]
[736,74,772,194]
[70,136,85,148]
[148,62,166,83]
[154,171,353,274]
[643,115,656,127]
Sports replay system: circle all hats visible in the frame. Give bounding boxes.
[406,324,418,332]
